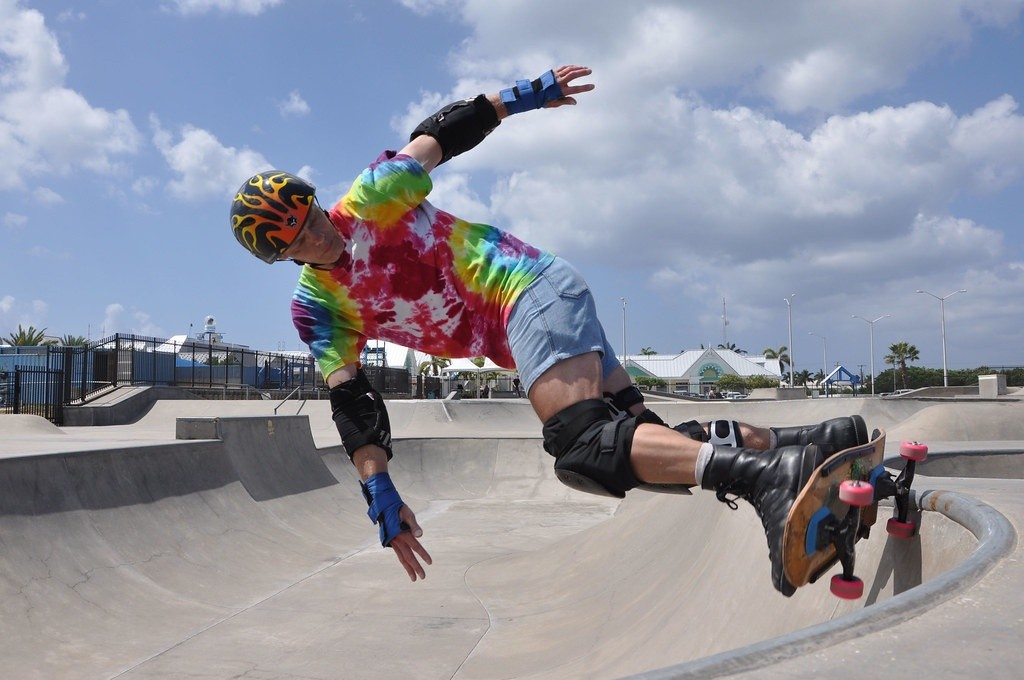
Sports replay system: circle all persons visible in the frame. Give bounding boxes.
[228,64,870,596]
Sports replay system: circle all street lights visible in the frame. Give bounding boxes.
[808,331,828,379]
[851,313,893,398]
[782,293,797,389]
[620,296,628,370]
[916,287,969,388]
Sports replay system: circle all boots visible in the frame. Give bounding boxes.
[769,415,870,463]
[699,440,820,600]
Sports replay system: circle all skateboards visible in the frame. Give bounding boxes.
[781,425,929,600]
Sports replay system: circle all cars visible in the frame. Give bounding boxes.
[880,389,915,396]
[674,387,747,400]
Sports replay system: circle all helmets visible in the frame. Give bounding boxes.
[230,170,317,266]
